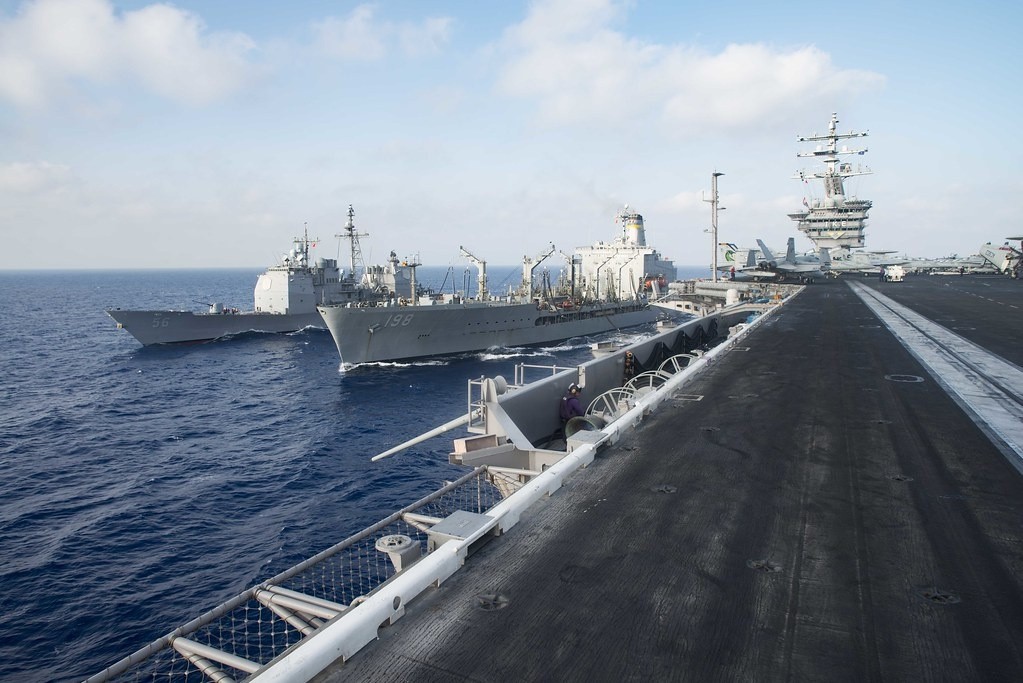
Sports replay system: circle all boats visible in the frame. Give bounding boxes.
[315,241,665,376]
[102,203,446,349]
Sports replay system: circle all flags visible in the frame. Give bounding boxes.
[312,243,315,248]
[802,196,810,210]
[402,261,406,267]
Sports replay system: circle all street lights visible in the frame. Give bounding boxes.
[711,172,725,283]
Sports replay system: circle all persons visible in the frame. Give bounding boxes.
[560,382,592,430]
[730,266,736,280]
[960,266,964,275]
[879,263,885,281]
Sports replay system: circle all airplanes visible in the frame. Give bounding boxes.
[734,231,1023,280]
